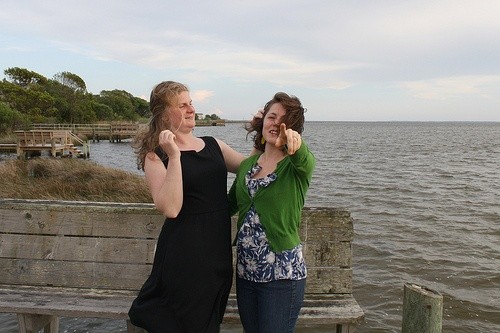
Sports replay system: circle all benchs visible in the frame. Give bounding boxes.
[0,198,365,333]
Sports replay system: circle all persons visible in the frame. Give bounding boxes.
[126,78,262,333]
[223,91,316,333]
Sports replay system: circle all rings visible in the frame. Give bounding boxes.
[293,135,299,141]
